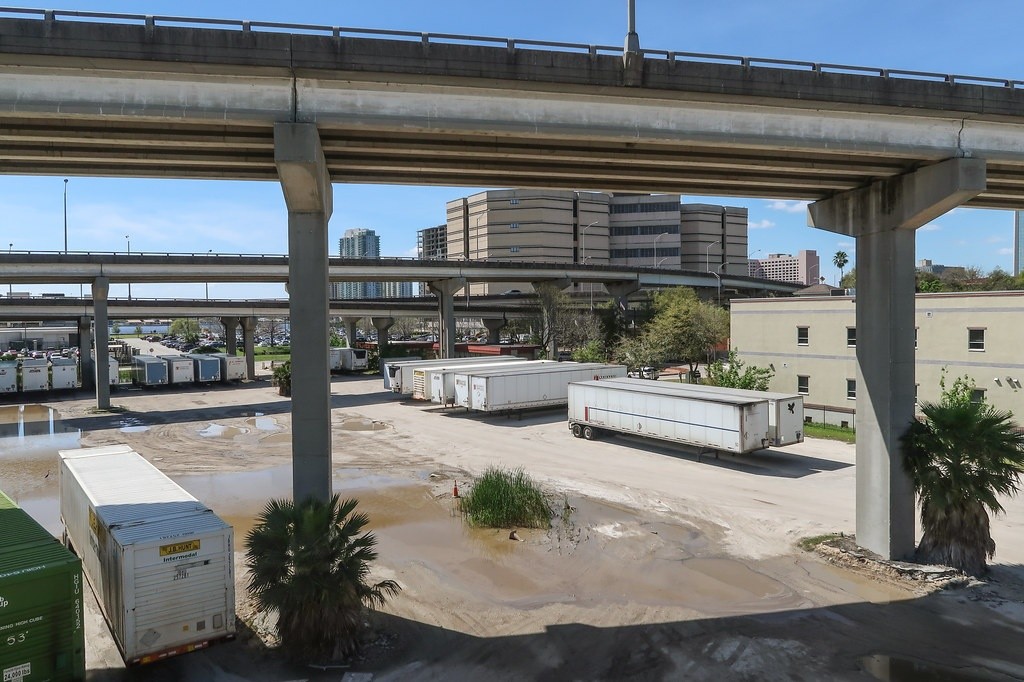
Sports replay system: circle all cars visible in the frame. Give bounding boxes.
[500,289,521,296]
[0,345,80,363]
[141,326,290,354]
[328,326,532,343]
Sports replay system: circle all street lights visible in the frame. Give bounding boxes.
[708,271,720,307]
[125,235,131,300]
[580,256,591,292]
[706,240,720,273]
[583,221,599,265]
[63,178,68,255]
[654,232,668,268]
[206,249,212,302]
[749,249,762,276]
[808,263,818,286]
[717,262,730,273]
[477,208,492,262]
[9,243,13,299]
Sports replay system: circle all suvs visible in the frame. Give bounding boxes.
[627,366,660,380]
